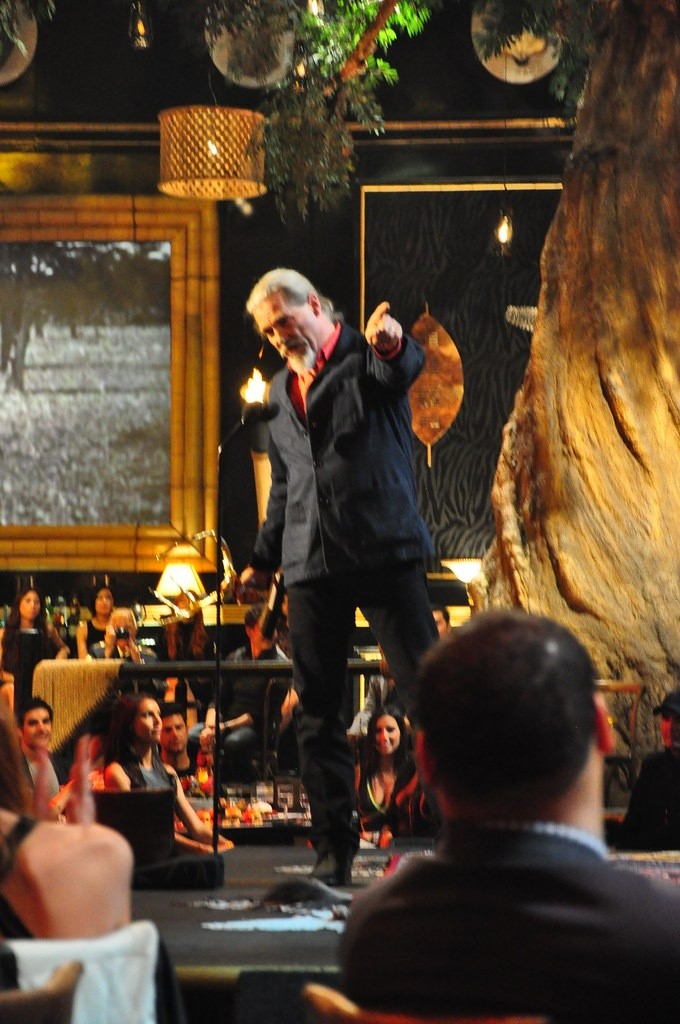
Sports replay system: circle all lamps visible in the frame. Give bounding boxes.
[156,75,269,200]
[157,563,209,618]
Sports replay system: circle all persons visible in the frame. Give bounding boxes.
[237,268,443,886]
[615,688,680,854]
[335,613,679,1024]
[1,587,432,1024]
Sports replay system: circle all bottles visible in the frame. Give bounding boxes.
[45,596,80,625]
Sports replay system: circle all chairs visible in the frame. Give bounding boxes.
[90,788,175,864]
[0,917,184,1024]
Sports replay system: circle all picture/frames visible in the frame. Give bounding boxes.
[1,193,222,571]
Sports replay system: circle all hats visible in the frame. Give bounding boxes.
[652,691,680,718]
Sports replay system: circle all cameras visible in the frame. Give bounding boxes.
[115,627,129,639]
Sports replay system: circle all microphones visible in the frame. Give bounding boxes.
[217,402,263,451]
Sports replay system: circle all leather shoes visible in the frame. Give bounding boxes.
[311,850,353,886]
